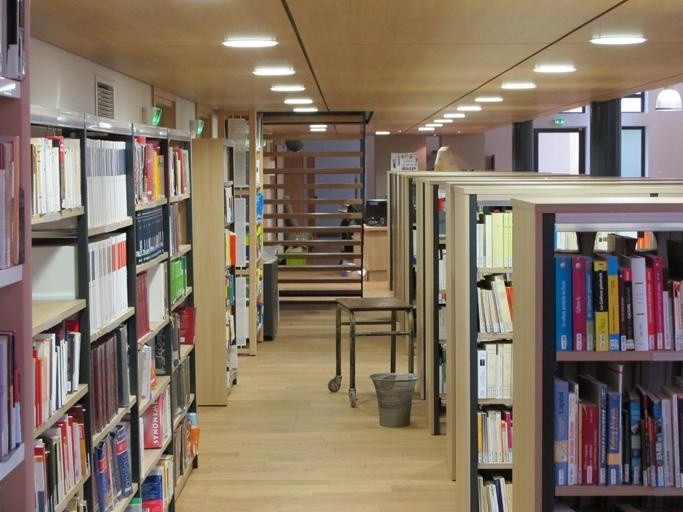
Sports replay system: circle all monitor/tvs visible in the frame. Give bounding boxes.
[366,199,388,225]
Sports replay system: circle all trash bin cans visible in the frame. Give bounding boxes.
[370,373,419,428]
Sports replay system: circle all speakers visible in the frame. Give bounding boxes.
[143,107,162,127]
[190,120,204,134]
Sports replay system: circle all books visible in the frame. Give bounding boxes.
[438,185,446,402]
[225,116,265,389]
[135,138,199,512]
[30,135,91,512]
[0,0,27,462]
[87,138,142,511]
[475,206,513,511]
[555,227,683,492]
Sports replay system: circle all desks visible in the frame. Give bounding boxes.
[353,226,388,281]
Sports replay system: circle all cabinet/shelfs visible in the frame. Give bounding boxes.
[285,151,317,240]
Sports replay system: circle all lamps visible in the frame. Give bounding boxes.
[500,82,537,91]
[309,124,328,132]
[269,82,306,92]
[473,96,504,105]
[284,97,312,104]
[375,130,390,135]
[222,32,278,48]
[533,62,576,73]
[417,104,483,132]
[293,107,318,112]
[654,86,682,112]
[252,65,296,76]
[588,32,648,45]
